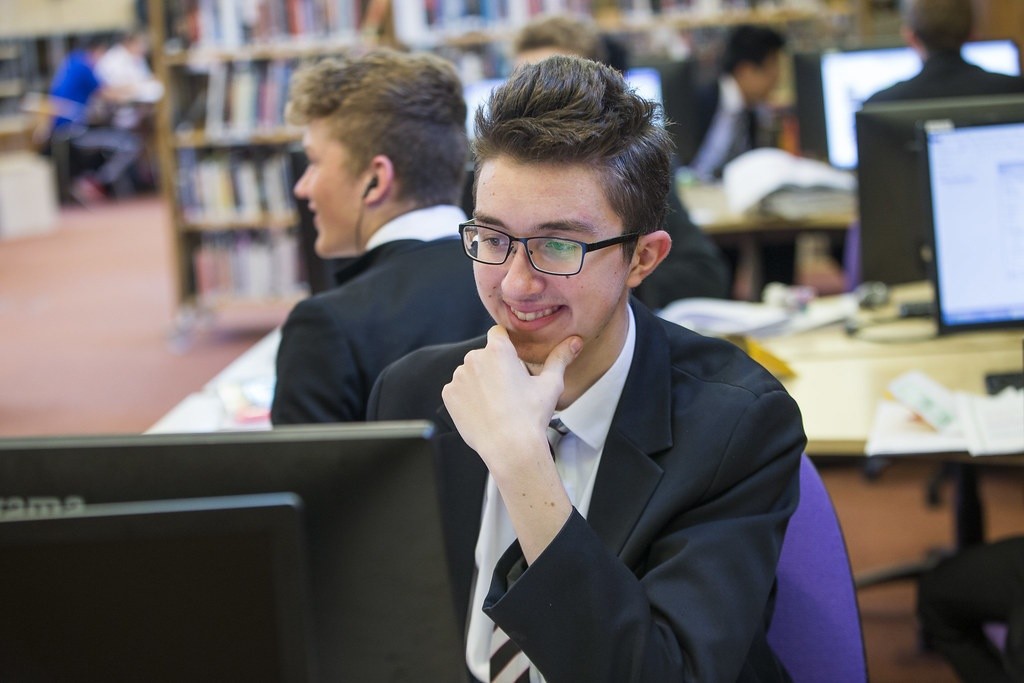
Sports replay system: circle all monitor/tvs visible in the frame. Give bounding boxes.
[854,94,1024,286]
[819,38,1022,170]
[0,419,465,683]
[917,116,1023,334]
[459,67,665,173]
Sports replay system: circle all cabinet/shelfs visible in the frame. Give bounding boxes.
[146,1,1024,325]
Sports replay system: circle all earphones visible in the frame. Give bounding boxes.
[363,175,379,198]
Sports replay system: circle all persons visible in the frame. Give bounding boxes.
[864,0,1024,104]
[95,27,154,125]
[50,33,139,206]
[520,13,722,307]
[272,54,496,426]
[367,55,807,683]
[659,23,787,176]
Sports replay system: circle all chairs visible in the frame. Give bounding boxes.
[761,455,867,679]
[21,94,91,208]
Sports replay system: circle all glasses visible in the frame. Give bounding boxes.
[459,219,639,276]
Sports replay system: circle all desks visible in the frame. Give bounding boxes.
[702,277,1024,595]
[669,188,856,302]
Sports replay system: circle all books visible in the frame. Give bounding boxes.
[172,0,769,304]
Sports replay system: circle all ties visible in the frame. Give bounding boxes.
[490,418,570,683]
